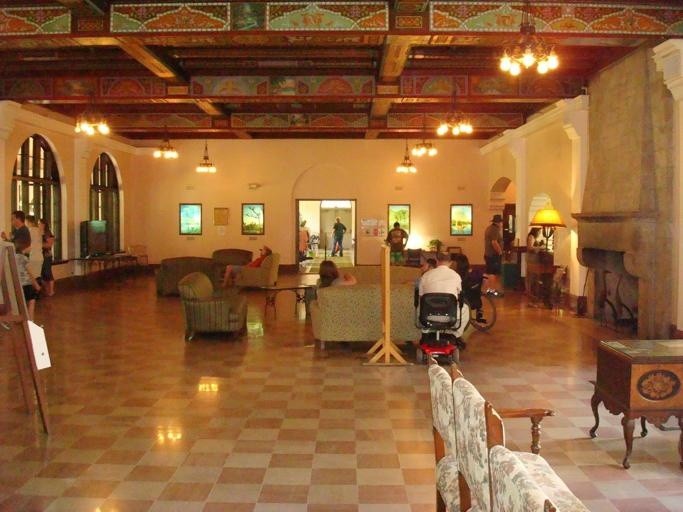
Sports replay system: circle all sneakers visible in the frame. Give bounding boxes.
[486,288,504,297]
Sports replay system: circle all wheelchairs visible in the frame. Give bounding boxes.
[460,268,501,331]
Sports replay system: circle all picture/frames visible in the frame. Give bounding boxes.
[179,203,202,235]
[241,203,265,236]
[450,204,473,236]
[388,204,410,235]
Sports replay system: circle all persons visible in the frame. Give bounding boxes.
[521,231,536,295]
[414,251,471,349]
[384,222,409,264]
[330,216,347,256]
[0,210,56,331]
[483,214,504,296]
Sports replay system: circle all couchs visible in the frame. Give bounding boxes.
[156,256,221,296]
[317,266,419,285]
[310,282,422,352]
[213,249,253,264]
[425,355,593,512]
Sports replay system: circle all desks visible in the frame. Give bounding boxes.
[588,339,683,469]
[72,254,137,288]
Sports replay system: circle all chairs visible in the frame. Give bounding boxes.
[236,253,280,288]
[176,272,247,338]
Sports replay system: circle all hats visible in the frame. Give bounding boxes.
[489,215,504,223]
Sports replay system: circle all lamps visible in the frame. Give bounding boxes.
[500,0,559,77]
[528,207,568,307]
[152,125,216,173]
[395,129,437,174]
[436,78,473,137]
[73,90,110,136]
